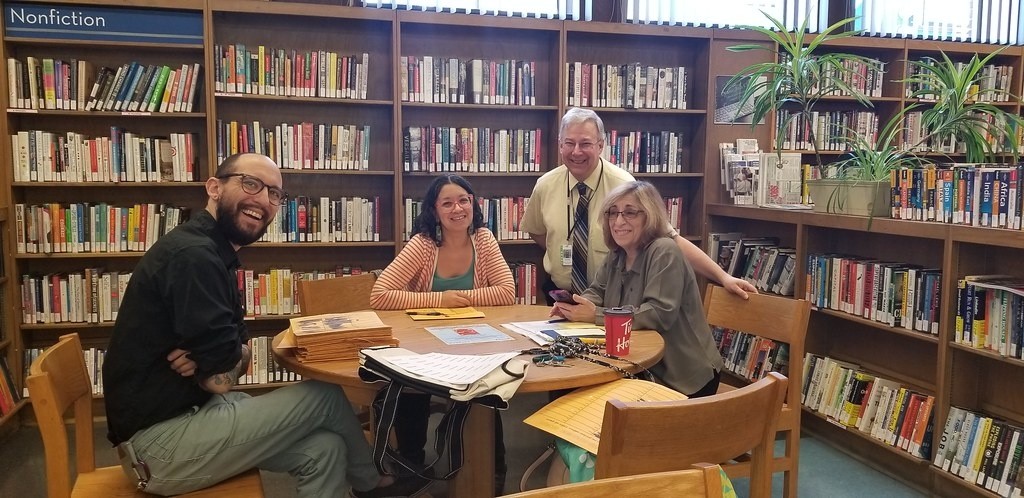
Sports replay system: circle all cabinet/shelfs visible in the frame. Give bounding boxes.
[0,0,1024,498]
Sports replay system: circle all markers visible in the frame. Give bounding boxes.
[533,356,564,362]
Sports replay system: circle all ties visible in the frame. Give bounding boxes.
[571,183,589,296]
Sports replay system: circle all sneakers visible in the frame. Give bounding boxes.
[348,466,438,498]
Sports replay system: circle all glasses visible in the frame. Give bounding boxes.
[434,194,473,214]
[605,208,644,220]
[562,138,602,152]
[218,173,288,206]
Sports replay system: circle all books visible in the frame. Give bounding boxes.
[8,53,1024,498]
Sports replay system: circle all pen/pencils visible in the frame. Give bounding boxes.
[405,312,445,315]
[548,319,566,323]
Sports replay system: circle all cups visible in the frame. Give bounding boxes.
[603,305,634,356]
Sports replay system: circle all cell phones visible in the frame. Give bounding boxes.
[549,289,578,305]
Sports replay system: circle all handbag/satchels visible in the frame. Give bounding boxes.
[519,436,738,498]
[357,343,529,481]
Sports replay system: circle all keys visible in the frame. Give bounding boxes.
[132,460,151,491]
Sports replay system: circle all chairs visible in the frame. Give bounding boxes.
[26,331,265,498]
[702,283,812,498]
[293,270,400,456]
[594,370,786,498]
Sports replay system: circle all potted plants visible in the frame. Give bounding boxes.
[719,3,1024,230]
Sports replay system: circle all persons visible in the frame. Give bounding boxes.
[550,181,725,400]
[370,174,515,496]
[102,153,434,498]
[519,107,757,403]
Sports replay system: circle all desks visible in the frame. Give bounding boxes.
[272,305,664,498]
